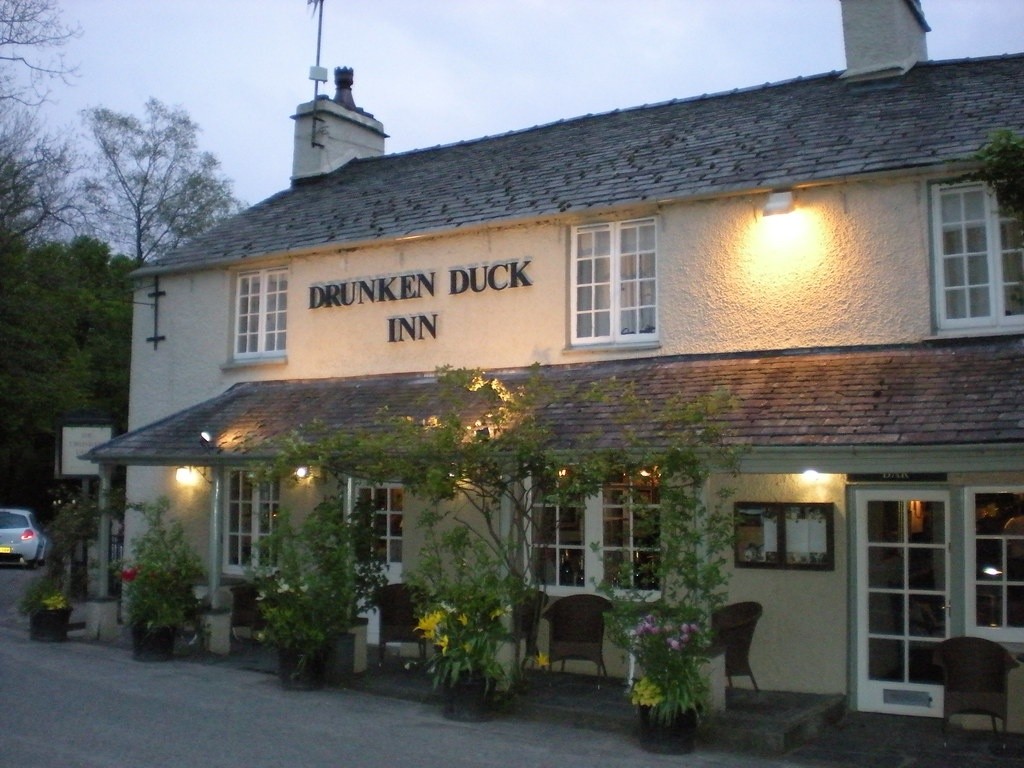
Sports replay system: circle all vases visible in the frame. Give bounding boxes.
[433,660,508,721]
[272,635,358,690]
[26,603,74,640]
[628,697,708,752]
[142,618,206,661]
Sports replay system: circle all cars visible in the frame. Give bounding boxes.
[0,508,48,570]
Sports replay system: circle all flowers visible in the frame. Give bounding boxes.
[603,608,733,725]
[254,493,388,644]
[399,510,552,687]
[120,499,214,640]
[17,496,102,615]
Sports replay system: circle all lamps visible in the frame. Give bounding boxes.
[198,432,213,453]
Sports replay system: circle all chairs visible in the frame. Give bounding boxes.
[711,600,763,696]
[372,580,614,681]
[229,581,279,648]
[919,635,1020,752]
